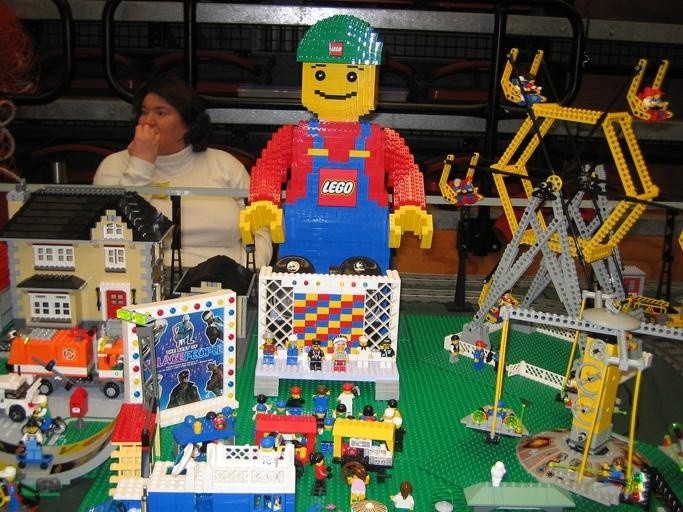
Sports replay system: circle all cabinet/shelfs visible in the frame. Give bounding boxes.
[0,0,683,236]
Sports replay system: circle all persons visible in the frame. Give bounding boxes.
[453,74,667,308]
[240,16,434,340]
[250,333,414,512]
[2,395,51,512]
[93,78,252,271]
[600,457,625,482]
[491,461,506,486]
[448,335,500,372]
